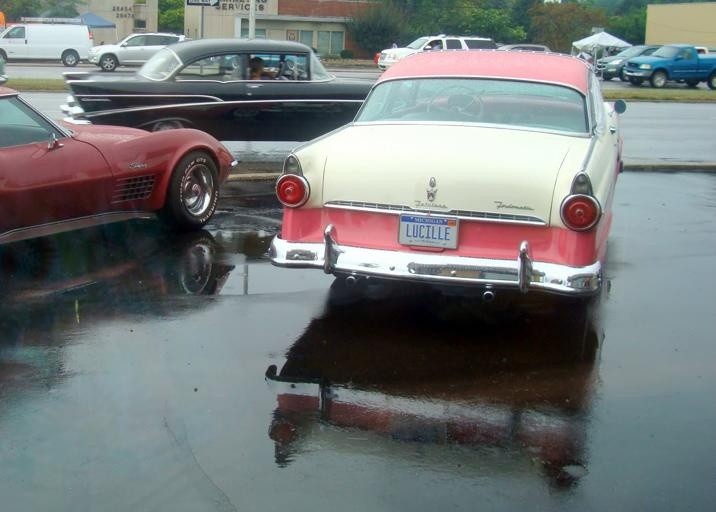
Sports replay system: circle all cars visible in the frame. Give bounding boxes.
[595,45,664,82]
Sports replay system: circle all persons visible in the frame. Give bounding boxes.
[248,56,300,80]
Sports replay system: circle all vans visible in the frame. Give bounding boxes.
[0,18,95,68]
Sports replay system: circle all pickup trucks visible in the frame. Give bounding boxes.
[623,44,716,89]
